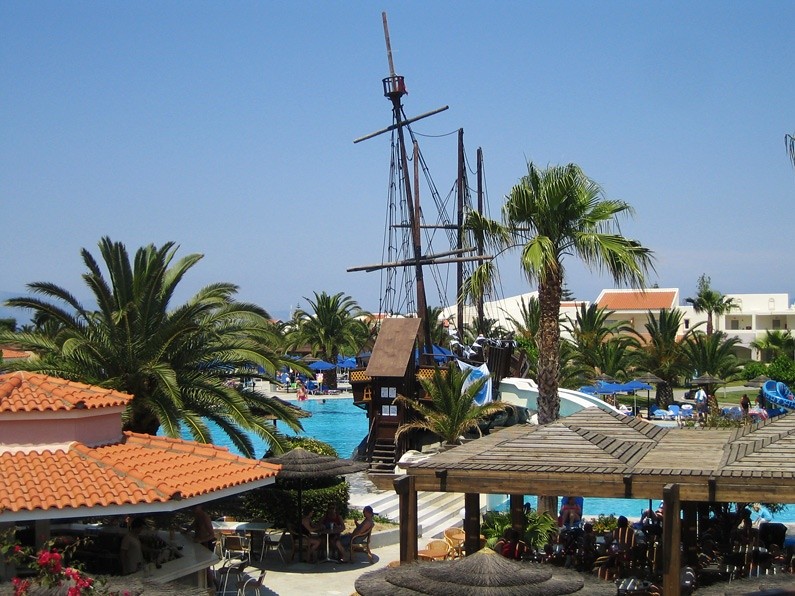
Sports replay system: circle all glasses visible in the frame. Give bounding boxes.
[328,507,336,510]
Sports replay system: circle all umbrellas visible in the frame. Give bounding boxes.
[591,373,623,402]
[258,345,456,383]
[630,372,666,402]
[747,374,774,382]
[260,446,372,563]
[578,379,653,416]
[686,371,725,384]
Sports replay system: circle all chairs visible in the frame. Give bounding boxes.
[426,528,486,561]
[650,403,769,423]
[216,515,375,596]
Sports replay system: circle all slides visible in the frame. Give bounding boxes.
[761,380,795,410]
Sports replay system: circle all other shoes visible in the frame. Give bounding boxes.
[336,560,347,564]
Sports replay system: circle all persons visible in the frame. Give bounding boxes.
[739,394,752,420]
[296,501,375,564]
[497,496,772,575]
[693,386,707,412]
[185,504,216,588]
[316,398,329,404]
[283,373,309,402]
[119,517,147,575]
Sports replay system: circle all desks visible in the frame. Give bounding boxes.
[417,550,449,561]
[212,521,268,563]
